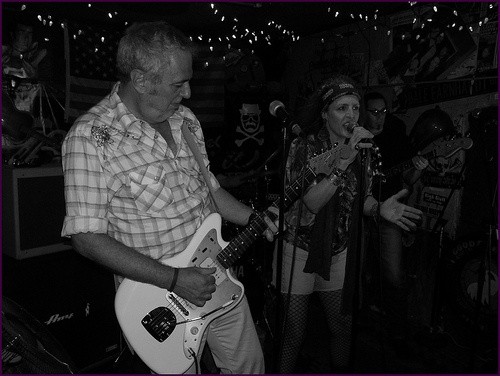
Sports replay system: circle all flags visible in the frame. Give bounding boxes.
[64,19,223,122]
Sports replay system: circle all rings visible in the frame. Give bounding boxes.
[276,231,279,235]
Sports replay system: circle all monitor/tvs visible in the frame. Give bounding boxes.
[0,165,72,259]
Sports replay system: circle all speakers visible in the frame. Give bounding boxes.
[3,257,122,372]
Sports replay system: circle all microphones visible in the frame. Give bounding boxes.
[269,100,306,140]
[347,122,381,158]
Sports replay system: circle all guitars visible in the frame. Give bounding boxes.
[2,91,61,152]
[113,142,350,376]
[381,136,474,184]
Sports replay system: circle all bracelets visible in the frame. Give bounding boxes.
[325,168,344,185]
[168,268,179,293]
[376,199,384,217]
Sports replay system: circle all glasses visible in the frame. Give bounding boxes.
[367,106,389,116]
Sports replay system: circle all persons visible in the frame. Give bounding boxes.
[269,76,423,374]
[359,92,429,306]
[62,21,285,376]
[1,18,63,166]
